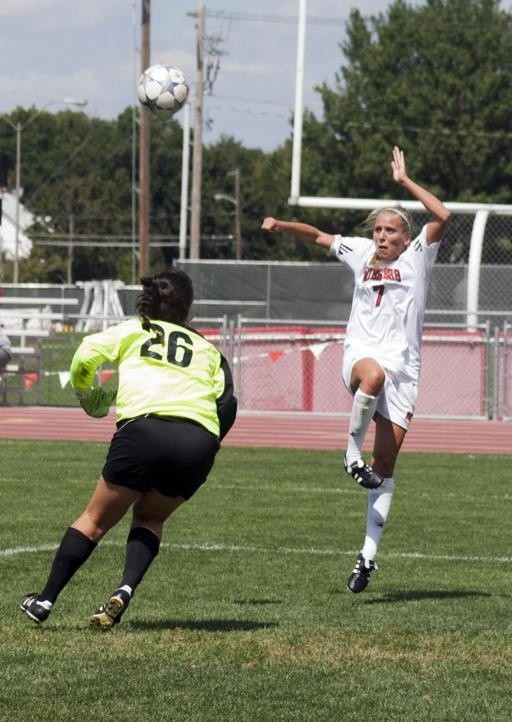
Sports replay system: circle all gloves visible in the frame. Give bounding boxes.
[80,387,117,418]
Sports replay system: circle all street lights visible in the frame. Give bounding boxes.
[0,97,88,283]
[214,169,240,260]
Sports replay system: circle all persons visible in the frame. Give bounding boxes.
[20,263,238,632]
[260,145,450,594]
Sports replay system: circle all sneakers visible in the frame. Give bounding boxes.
[346,553,378,593]
[343,452,383,489]
[19,592,51,624]
[89,587,130,633]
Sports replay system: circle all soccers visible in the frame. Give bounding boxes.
[136,64,188,119]
